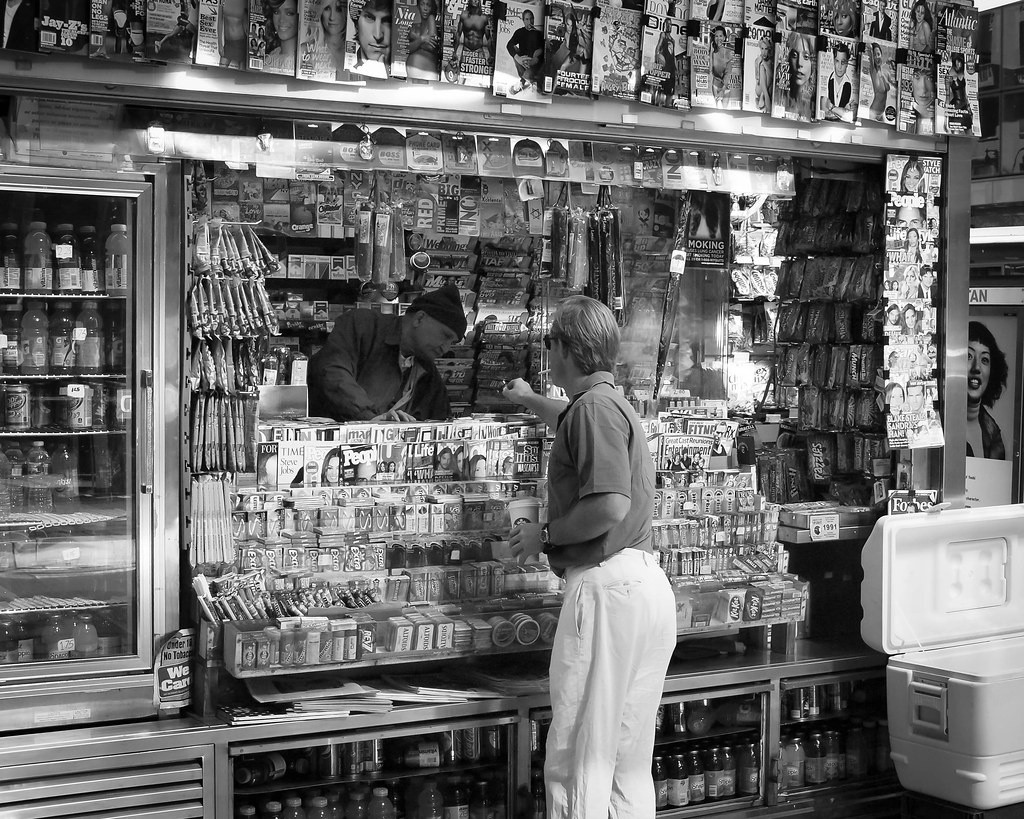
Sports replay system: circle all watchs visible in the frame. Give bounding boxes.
[540,523,551,550]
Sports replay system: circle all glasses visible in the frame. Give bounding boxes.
[542,334,556,350]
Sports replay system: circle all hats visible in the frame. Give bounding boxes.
[405,283,467,344]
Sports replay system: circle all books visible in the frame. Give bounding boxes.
[881,153,946,451]
[1,0,986,141]
[257,413,741,502]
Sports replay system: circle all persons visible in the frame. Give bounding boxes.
[502,296,677,817]
[967,320,1010,461]
[305,282,468,423]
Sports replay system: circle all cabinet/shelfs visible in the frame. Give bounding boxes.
[228,649,905,819]
[0,158,183,736]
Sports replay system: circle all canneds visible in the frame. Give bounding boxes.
[5,383,30,431]
[66,388,111,429]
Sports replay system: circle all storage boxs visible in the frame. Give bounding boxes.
[861,502,1024,812]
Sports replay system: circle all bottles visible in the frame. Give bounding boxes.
[235,755,318,783]
[651,716,894,811]
[1,206,135,664]
[532,773,546,819]
[239,772,510,819]
